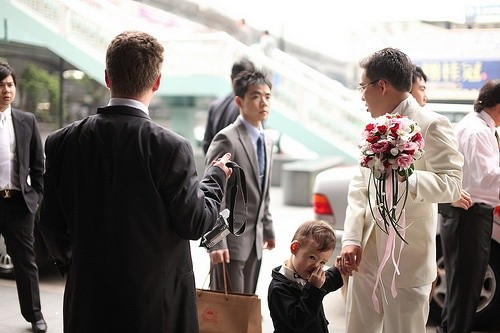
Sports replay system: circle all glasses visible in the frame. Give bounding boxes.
[357,79,387,94]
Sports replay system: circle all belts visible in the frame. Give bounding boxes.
[0,189,22,198]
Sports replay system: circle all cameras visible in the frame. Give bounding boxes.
[200,209,233,250]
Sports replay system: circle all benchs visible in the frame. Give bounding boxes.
[272,153,302,186]
[281,159,346,208]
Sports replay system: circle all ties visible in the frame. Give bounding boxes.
[257,134,265,188]
[495,130,500,166]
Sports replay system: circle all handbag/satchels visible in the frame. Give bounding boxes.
[196,262,262,333]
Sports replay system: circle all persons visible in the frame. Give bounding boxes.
[404,66,500,333]
[0,62,47,333]
[340,47,463,333]
[268,221,357,333]
[203,57,275,296]
[40,33,232,333]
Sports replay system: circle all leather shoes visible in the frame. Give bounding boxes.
[32,319,47,333]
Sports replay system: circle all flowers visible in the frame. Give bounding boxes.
[358,113,426,314]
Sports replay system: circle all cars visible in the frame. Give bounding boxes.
[312,165,500,331]
